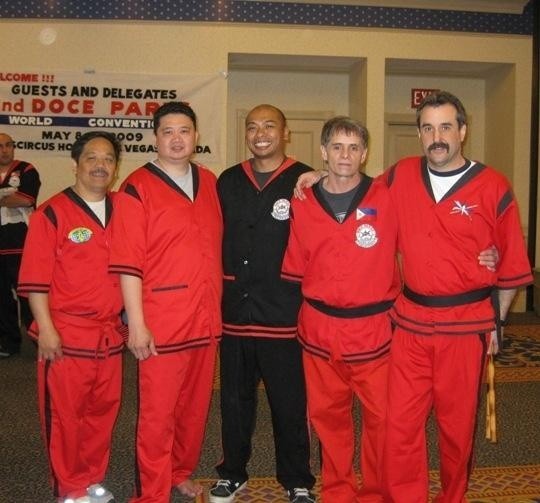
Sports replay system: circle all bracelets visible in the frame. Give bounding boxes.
[498,318,509,327]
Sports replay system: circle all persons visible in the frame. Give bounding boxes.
[277,112,405,502]
[203,100,318,503]
[0,131,42,358]
[291,87,536,502]
[16,129,122,503]
[106,100,227,501]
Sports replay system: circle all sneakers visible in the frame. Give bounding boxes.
[206,476,250,502]
[287,483,316,503]
[59,483,113,503]
